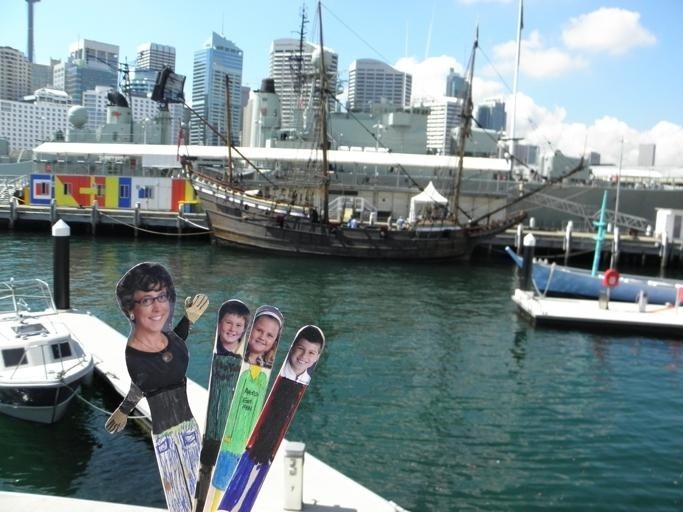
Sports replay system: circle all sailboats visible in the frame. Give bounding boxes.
[151,0,589,265]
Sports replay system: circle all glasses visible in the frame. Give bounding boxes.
[131,293,170,305]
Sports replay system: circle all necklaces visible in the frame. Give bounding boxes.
[130,331,173,364]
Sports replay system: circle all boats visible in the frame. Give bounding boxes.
[504,190,683,304]
[0,279,94,425]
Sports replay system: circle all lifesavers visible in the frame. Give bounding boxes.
[604,269,620,289]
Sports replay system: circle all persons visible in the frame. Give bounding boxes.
[114,259,190,433]
[244,302,283,370]
[216,298,252,355]
[301,202,450,234]
[278,323,326,387]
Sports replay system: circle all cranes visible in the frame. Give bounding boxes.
[86,49,132,109]
